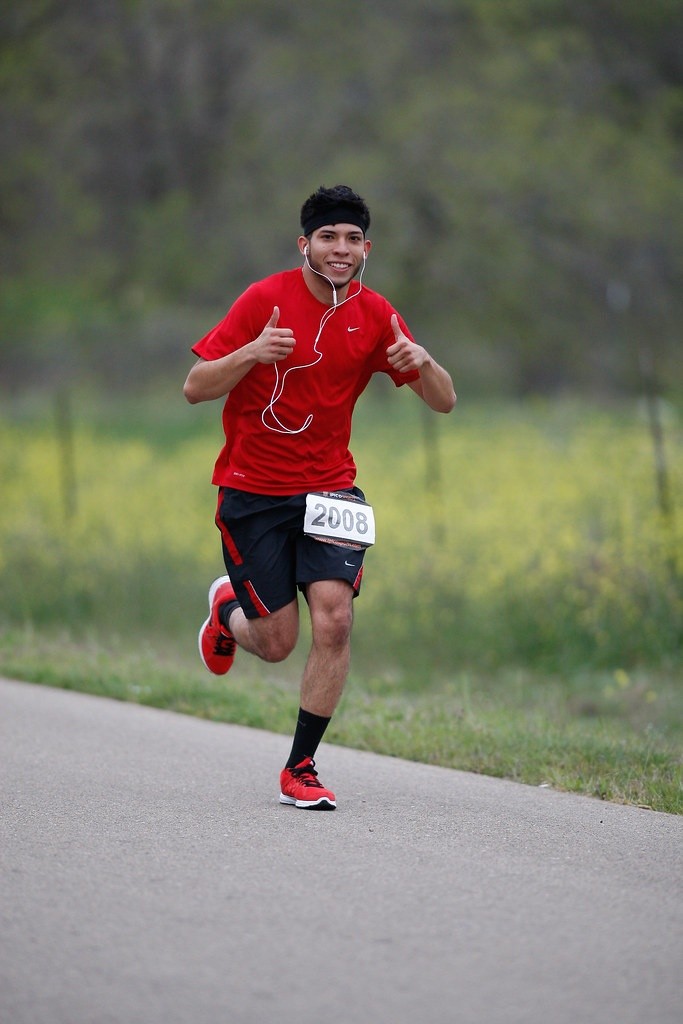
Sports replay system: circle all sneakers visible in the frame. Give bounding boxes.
[199,575,245,675]
[281,757,338,809]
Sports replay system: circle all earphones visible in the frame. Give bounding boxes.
[303,244,308,258]
[363,251,365,260]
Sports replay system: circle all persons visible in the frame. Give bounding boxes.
[184,185,457,811]
